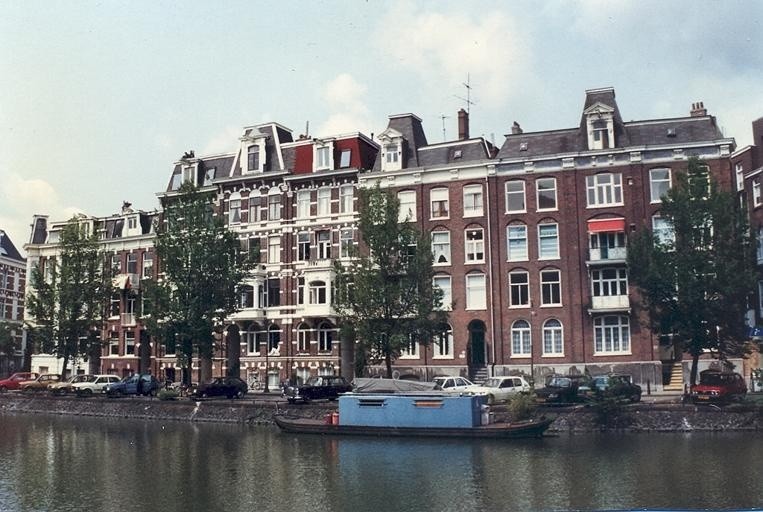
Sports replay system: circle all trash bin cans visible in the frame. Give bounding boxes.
[324,413,339,424]
[481,412,494,426]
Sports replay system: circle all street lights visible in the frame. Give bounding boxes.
[529,309,538,388]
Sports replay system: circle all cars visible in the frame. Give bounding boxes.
[690,372,747,405]
[0,372,159,398]
[432,376,531,404]
[533,374,642,407]
[194,376,248,398]
[280,375,354,403]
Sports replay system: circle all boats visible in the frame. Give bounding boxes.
[272,393,549,439]
